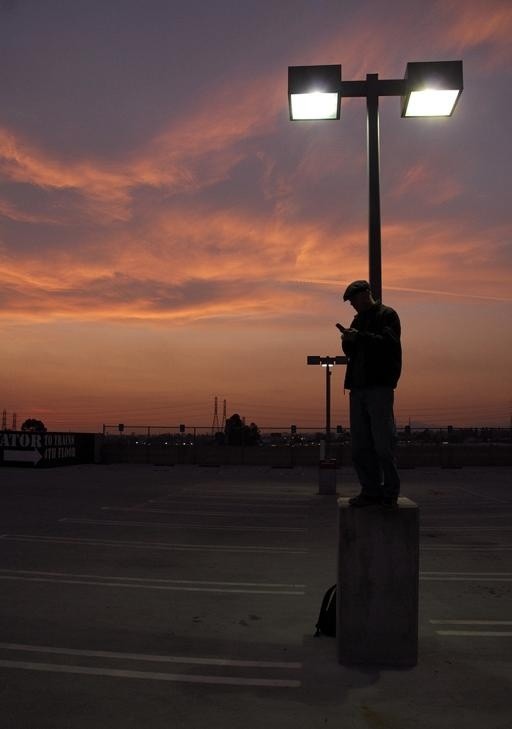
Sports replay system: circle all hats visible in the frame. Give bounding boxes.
[343,280,371,303]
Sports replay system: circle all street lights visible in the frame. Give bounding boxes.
[306,355,350,459]
[287,58,465,499]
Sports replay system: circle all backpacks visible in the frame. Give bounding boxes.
[313,584,337,639]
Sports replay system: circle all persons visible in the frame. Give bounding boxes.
[338,279,405,506]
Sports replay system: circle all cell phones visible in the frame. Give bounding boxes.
[336,323,346,332]
